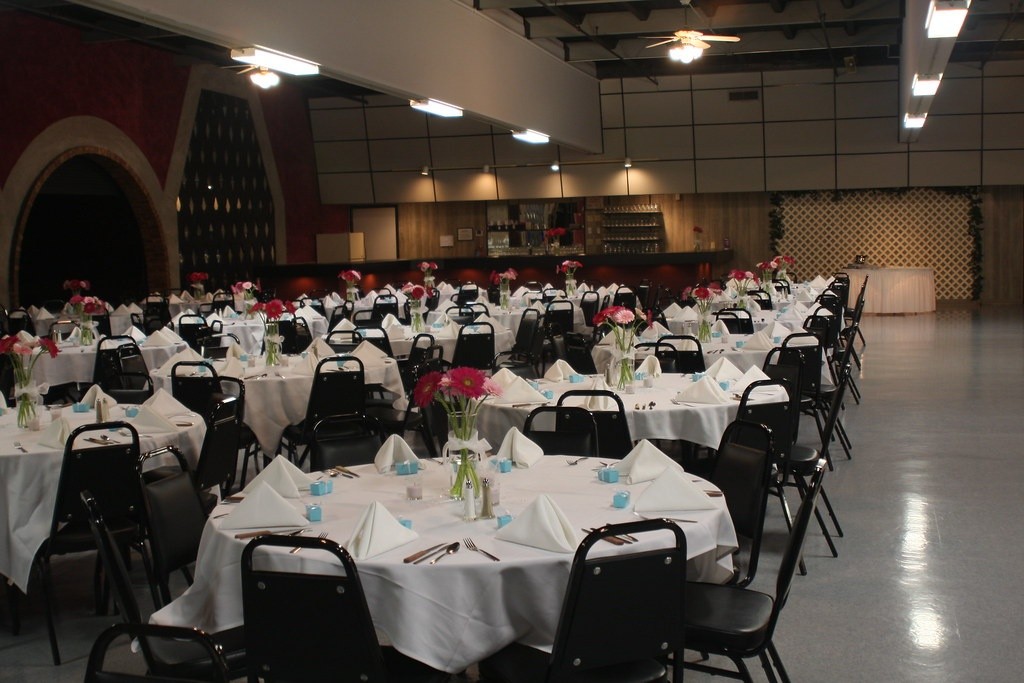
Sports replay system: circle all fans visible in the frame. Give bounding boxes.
[639,0,741,48]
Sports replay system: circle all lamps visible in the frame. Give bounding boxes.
[903,0,974,129]
[230,46,634,177]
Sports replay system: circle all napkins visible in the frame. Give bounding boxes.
[218,480,310,528]
[494,492,580,553]
[348,500,418,561]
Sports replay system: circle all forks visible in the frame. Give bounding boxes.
[13,441,27,453]
[463,537,500,562]
[566,457,588,465]
[290,532,328,554]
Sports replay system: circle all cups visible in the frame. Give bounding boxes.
[135,299,527,371]
[305,456,629,529]
[28,405,140,431]
[525,291,794,391]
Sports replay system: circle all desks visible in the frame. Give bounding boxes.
[129,449,738,683]
[591,334,834,385]
[149,356,410,458]
[466,367,786,462]
[1,402,208,597]
[664,308,814,333]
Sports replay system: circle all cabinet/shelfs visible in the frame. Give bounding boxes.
[601,210,664,254]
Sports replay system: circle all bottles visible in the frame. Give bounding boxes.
[52,329,62,344]
[96,398,110,423]
[486,202,662,255]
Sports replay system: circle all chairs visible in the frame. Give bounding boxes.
[0,272,869,683]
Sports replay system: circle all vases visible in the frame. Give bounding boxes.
[692,305,712,343]
[605,337,636,392]
[443,411,481,501]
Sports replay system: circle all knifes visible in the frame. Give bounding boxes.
[235,529,307,538]
[404,543,449,564]
[83,437,111,445]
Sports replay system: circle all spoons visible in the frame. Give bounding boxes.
[648,401,655,410]
[600,462,619,466]
[429,542,460,565]
[100,435,121,443]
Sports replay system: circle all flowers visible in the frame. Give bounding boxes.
[593,306,654,388]
[682,281,723,341]
[414,366,503,497]
